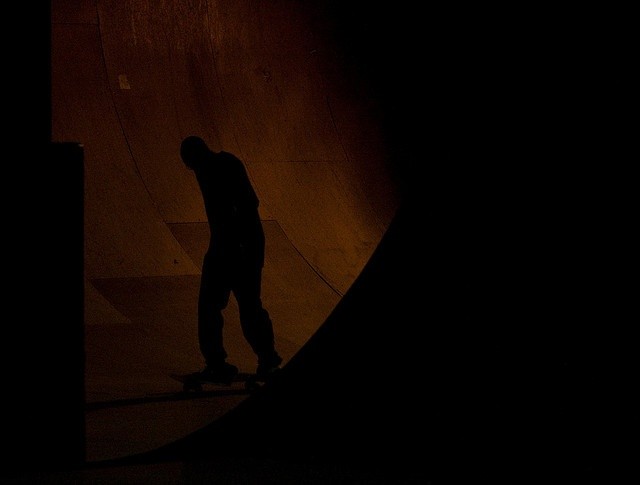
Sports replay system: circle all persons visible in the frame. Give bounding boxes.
[180,136,283,381]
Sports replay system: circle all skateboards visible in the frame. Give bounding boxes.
[170,373,276,392]
